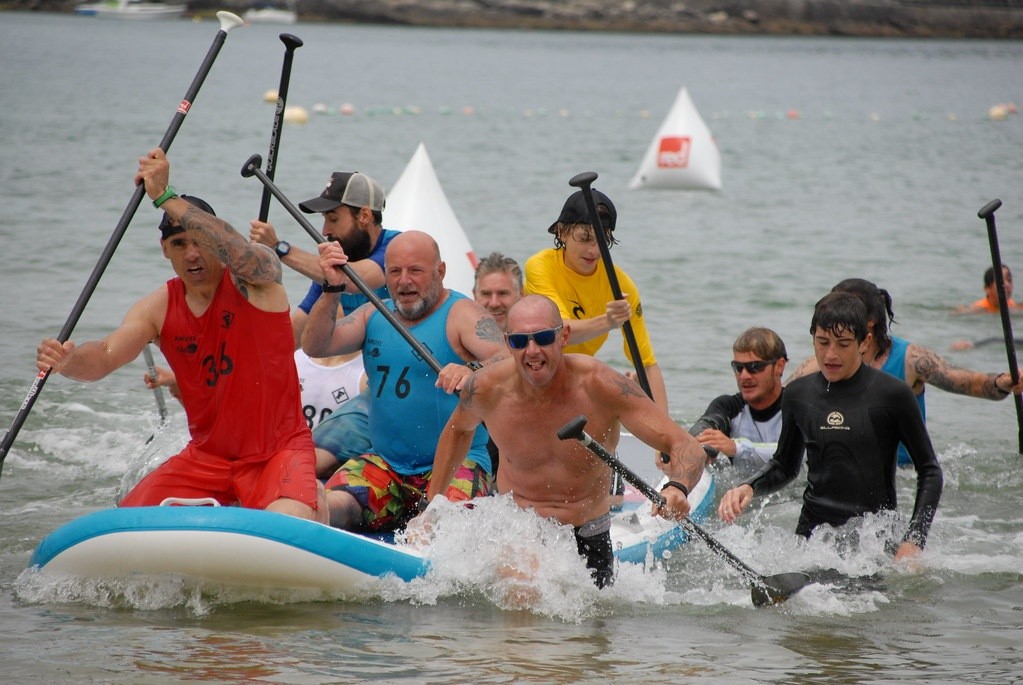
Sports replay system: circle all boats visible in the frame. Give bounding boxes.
[28,431,717,600]
[74,1,187,22]
[242,7,296,26]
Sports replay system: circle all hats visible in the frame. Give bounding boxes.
[548,187,617,234]
[298,171,384,214]
[158,193,216,239]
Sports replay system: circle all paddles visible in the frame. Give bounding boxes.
[977,198,1023,455]
[0,11,245,476]
[557,415,812,609]
[143,345,169,425]
[568,171,671,463]
[240,153,488,432]
[258,32,305,222]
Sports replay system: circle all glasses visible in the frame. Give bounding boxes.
[506,325,563,350]
[731,359,776,375]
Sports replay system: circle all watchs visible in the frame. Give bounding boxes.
[322,279,346,294]
[153,184,177,209]
[274,241,291,258]
[466,360,483,371]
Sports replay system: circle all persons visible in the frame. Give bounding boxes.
[784,278,1023,470]
[141,173,808,591]
[719,293,944,559]
[35,148,331,527]
[970,266,1018,314]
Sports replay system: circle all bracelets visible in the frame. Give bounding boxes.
[994,372,1010,394]
[662,481,688,498]
